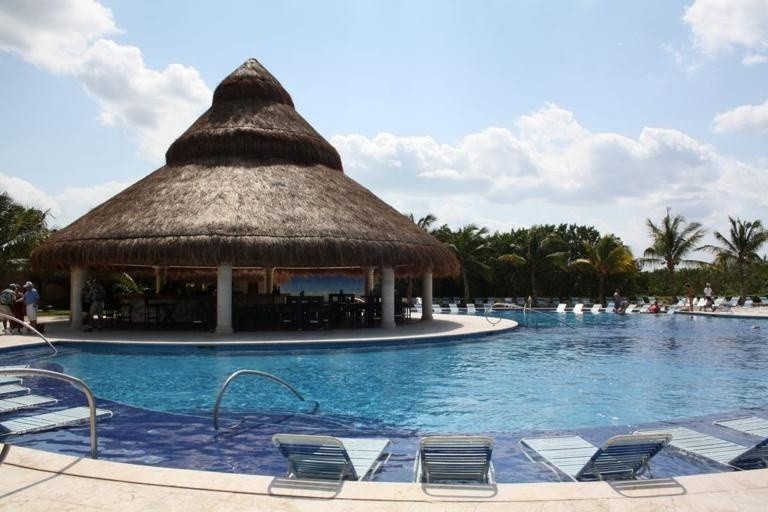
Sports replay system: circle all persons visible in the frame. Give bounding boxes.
[15,281,41,335]
[82,279,105,332]
[0,283,17,335]
[10,284,27,335]
[612,282,714,316]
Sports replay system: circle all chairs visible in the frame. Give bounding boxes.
[515,432,675,483]
[414,294,768,314]
[272,433,395,481]
[0,405,114,438]
[713,416,768,440]
[409,432,500,485]
[631,424,767,475]
